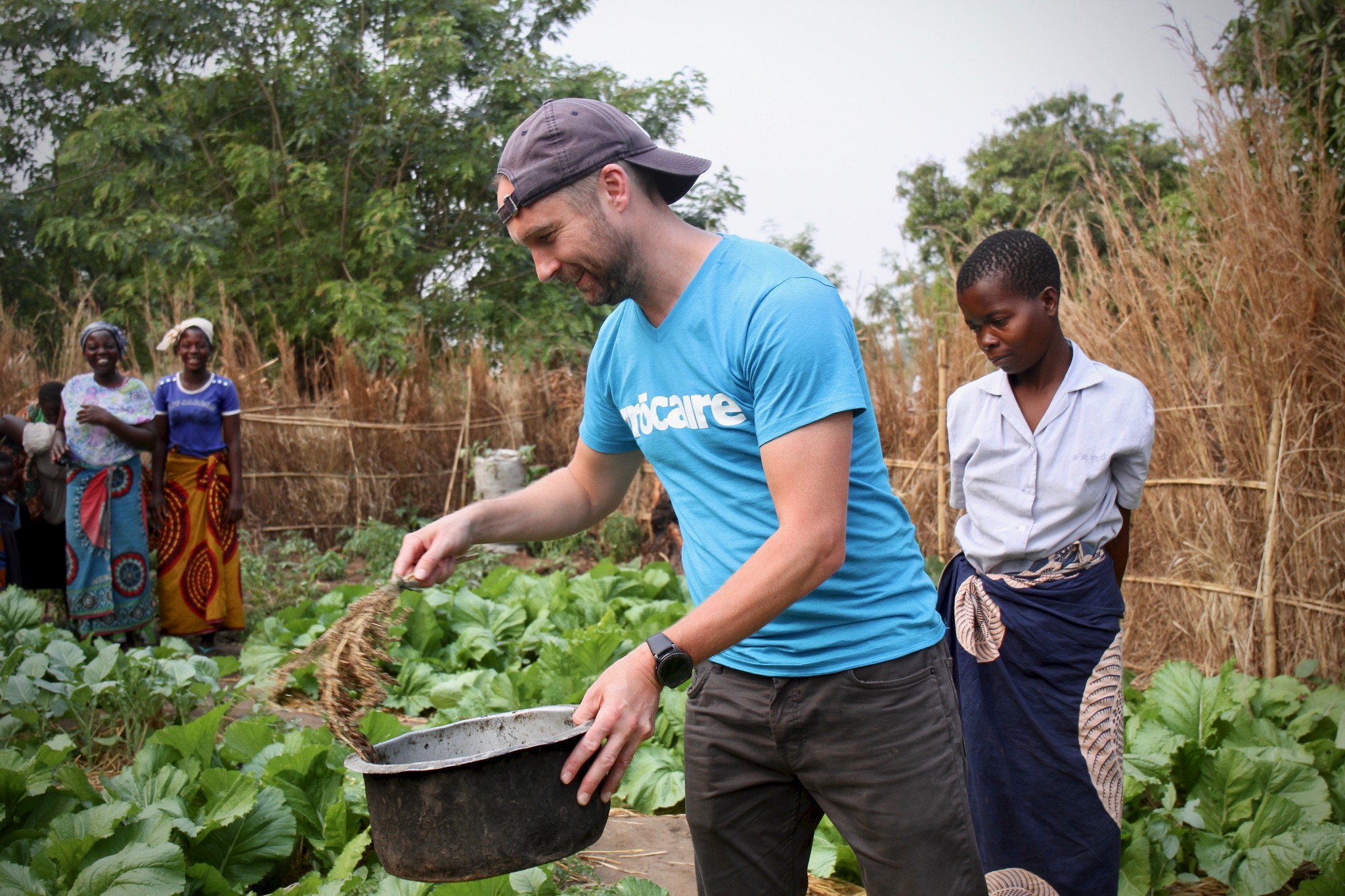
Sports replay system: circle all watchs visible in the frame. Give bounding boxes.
[646,632,695,689]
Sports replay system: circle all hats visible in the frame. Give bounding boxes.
[495,97,712,225]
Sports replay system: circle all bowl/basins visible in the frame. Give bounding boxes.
[345,704,610,884]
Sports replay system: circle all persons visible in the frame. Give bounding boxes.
[1,413,56,589]
[390,95,989,896]
[0,451,23,592]
[146,316,247,659]
[933,229,1155,895]
[12,381,71,590]
[49,321,161,654]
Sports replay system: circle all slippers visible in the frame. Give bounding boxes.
[198,639,218,657]
